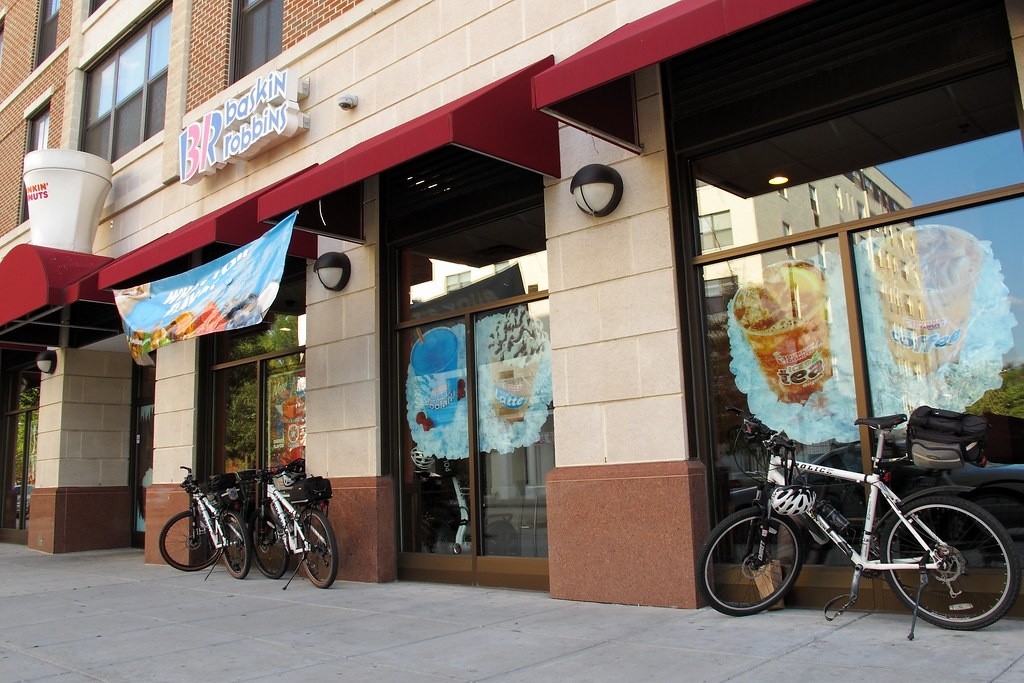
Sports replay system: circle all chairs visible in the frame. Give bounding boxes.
[451,477,513,554]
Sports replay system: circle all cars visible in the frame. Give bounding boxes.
[728,442,1024,555]
[13,484,36,519]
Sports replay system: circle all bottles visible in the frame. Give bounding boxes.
[284,513,296,538]
[814,498,859,543]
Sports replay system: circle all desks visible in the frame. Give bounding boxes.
[518,485,547,557]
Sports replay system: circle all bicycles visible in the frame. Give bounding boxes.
[158,465,251,581]
[693,413,1020,640]
[236,458,339,591]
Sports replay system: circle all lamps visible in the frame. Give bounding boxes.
[36,350,57,374]
[314,251,351,291]
[571,163,624,218]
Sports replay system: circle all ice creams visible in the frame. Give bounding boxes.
[487,304,549,423]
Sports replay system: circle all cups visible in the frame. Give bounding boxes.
[490,356,538,422]
[281,399,305,464]
[732,260,832,404]
[23,149,113,254]
[872,224,983,376]
[411,327,458,425]
